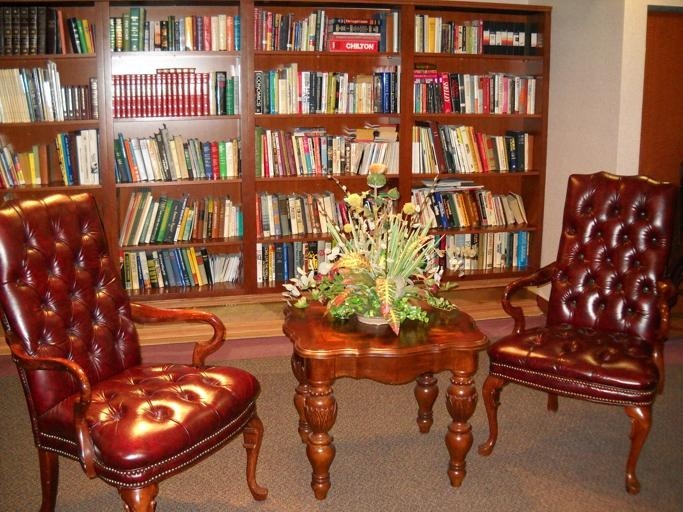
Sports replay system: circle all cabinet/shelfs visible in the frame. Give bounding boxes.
[0,0,552,309]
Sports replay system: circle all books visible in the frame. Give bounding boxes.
[1,7,100,187]
[107,7,244,289]
[254,7,400,284]
[410,14,538,271]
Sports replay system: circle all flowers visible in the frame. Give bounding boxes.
[281,163,479,336]
